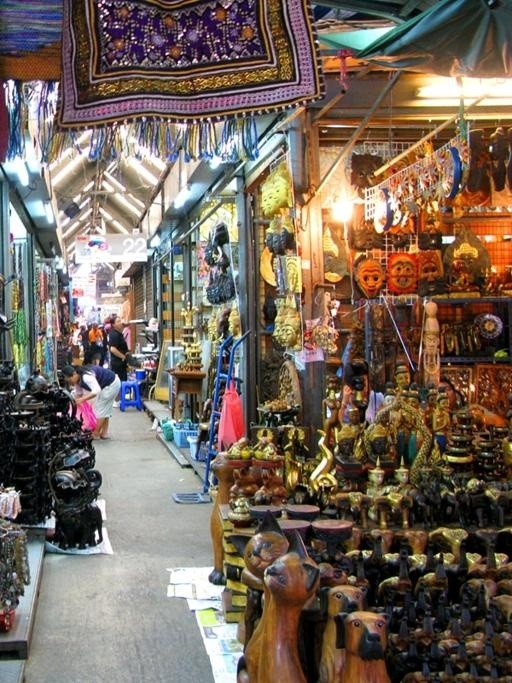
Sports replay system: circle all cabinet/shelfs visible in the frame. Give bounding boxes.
[154,338,184,400]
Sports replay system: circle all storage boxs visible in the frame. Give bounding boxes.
[187,435,199,459]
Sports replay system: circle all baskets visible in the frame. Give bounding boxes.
[160,417,206,461]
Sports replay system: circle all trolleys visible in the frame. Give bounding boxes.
[169,327,255,506]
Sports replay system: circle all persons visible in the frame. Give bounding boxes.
[425,331,442,385]
[393,363,412,389]
[200,167,307,352]
[326,357,390,422]
[437,376,462,409]
[77,302,132,388]
[382,393,394,404]
[431,388,450,449]
[60,364,122,438]
[422,386,437,421]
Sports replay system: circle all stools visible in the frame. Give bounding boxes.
[120,380,142,411]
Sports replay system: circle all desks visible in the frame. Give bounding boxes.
[165,367,206,424]
